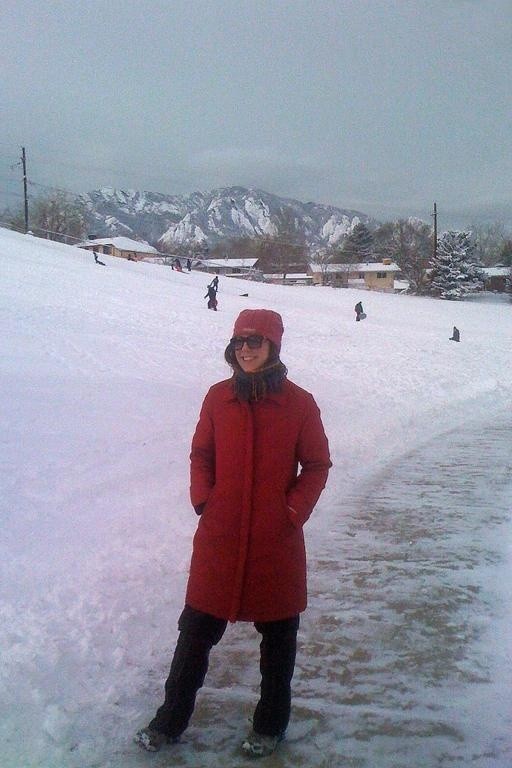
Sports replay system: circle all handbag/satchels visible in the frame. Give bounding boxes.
[358,313,367,320]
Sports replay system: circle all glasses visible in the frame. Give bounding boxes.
[229,337,272,350]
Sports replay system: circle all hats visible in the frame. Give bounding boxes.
[233,308,285,349]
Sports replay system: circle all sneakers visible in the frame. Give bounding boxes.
[240,730,285,757]
[133,724,180,752]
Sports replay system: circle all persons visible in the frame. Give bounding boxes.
[203,284,219,312]
[353,300,365,322]
[92,251,99,264]
[131,307,334,757]
[167,257,192,272]
[448,325,461,342]
[210,275,220,293]
